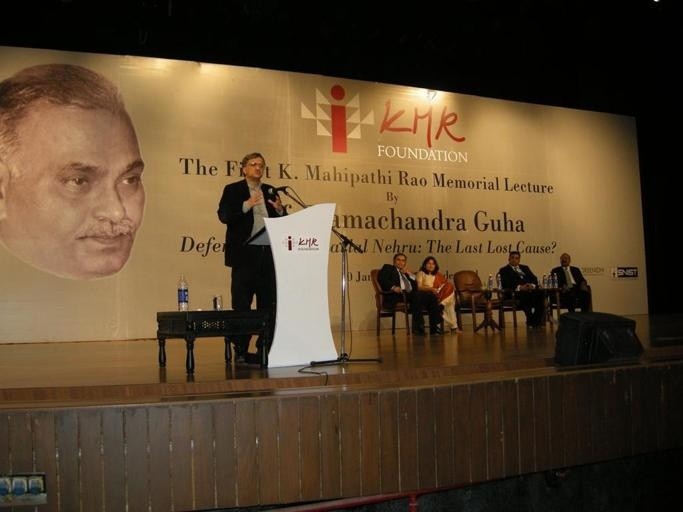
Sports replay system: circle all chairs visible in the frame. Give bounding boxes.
[454,270,504,333]
[370,269,423,335]
[495,273,548,329]
[414,271,444,335]
[548,285,593,329]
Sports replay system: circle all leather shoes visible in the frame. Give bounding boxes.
[430,327,448,334]
[235,350,249,363]
[414,328,424,335]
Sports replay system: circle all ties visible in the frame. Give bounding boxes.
[515,267,525,276]
[565,267,573,288]
[399,269,410,292]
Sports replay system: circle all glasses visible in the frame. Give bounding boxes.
[246,162,264,167]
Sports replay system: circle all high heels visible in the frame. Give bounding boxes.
[450,326,463,334]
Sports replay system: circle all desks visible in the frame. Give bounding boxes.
[157,310,271,370]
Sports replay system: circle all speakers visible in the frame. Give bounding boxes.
[556,313,645,366]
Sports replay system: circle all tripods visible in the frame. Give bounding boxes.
[284,191,383,365]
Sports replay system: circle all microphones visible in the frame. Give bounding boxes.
[268,186,288,194]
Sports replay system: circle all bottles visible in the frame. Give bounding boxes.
[542,273,558,289]
[488,274,502,290]
[177,275,189,312]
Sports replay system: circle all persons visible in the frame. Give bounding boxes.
[549,252,589,312]
[215,152,289,362]
[498,251,545,328]
[413,256,463,334]
[0,64,145,282]
[376,251,441,336]
